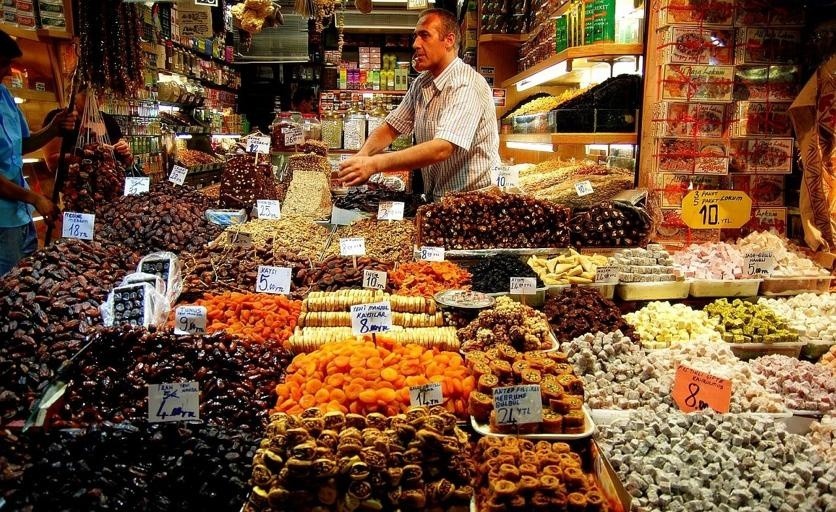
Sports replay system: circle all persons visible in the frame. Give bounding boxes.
[338,7,503,202]
[290,78,314,113]
[41,62,131,210]
[0,29,78,276]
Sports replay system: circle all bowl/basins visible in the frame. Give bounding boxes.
[205,208,248,227]
[432,289,497,311]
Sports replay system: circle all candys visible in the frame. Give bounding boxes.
[560,229,836,512]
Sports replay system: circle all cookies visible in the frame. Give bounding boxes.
[465,344,586,434]
[292,290,459,354]
[291,289,459,357]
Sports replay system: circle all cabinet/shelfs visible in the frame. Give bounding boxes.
[99,29,243,183]
[498,0,644,157]
[0,1,79,248]
[317,66,415,118]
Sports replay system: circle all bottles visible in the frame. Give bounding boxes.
[95,86,161,136]
[130,137,164,173]
[156,72,205,107]
[165,41,242,91]
[270,110,414,151]
[380,53,396,92]
[618,12,639,42]
[478,1,530,36]
